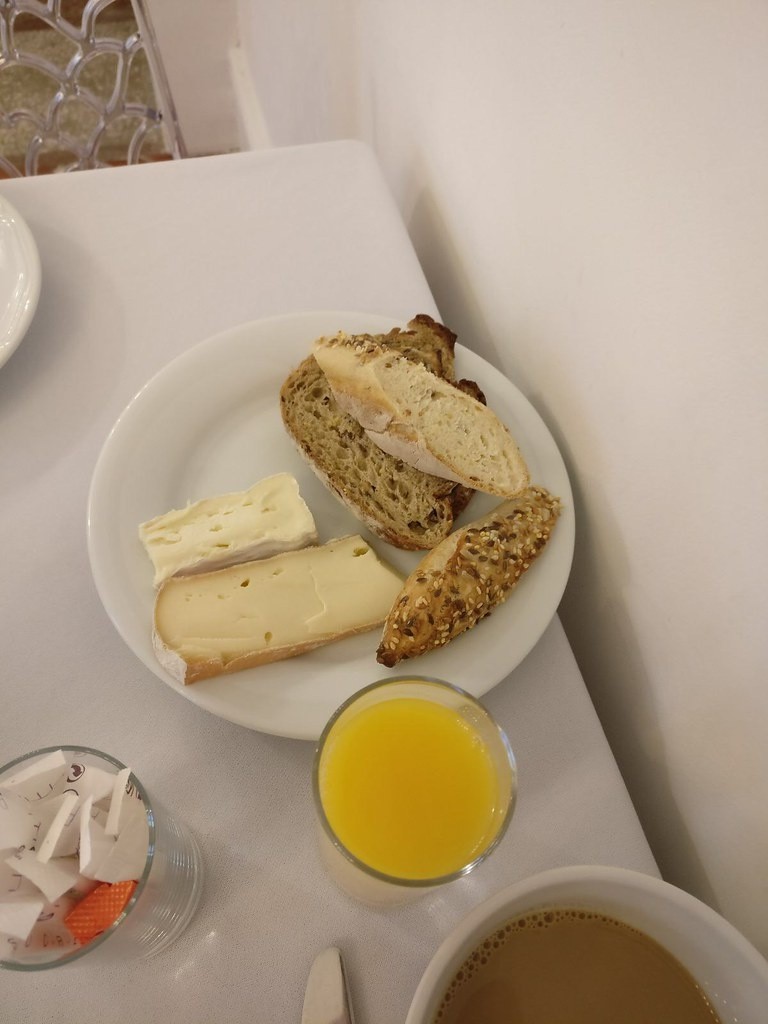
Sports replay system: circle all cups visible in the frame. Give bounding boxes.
[0,745,206,974]
[310,674,526,906]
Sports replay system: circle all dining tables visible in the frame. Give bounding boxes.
[0,139,663,1023]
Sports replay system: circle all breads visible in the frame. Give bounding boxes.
[136,312,561,686]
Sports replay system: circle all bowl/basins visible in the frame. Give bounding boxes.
[405,861,768,1024]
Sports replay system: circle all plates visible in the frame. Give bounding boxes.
[0,193,42,372]
[81,310,581,746]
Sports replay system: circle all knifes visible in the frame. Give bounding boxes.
[298,945,354,1023]
